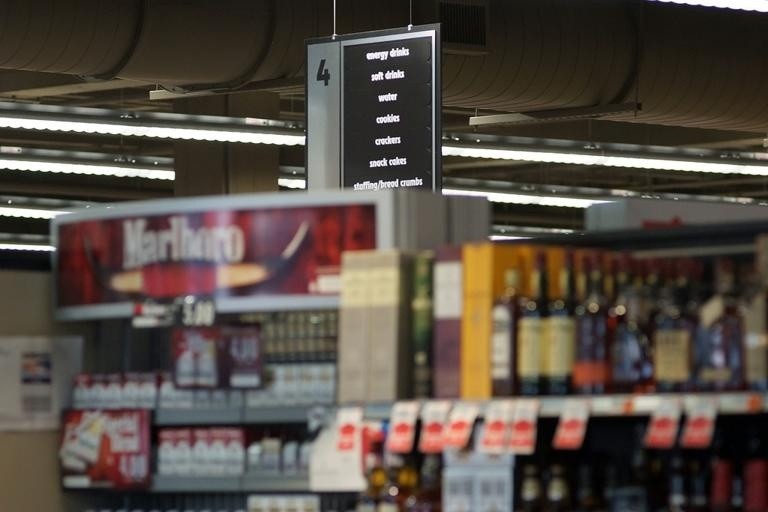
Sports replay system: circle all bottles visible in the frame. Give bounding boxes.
[489,249,768,395]
[260,312,339,405]
[357,425,768,512]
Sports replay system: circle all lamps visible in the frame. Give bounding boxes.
[276,165,308,191]
[489,223,577,241]
[0,146,175,186]
[0,194,114,222]
[0,102,305,151]
[439,130,768,180]
[441,177,761,211]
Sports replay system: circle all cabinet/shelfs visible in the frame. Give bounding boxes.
[57,311,356,512]
[332,220,768,512]
